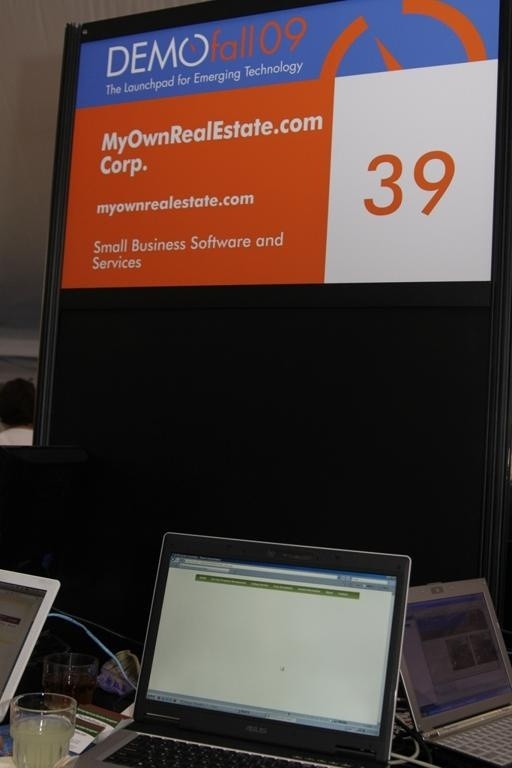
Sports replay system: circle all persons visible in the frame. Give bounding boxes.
[0,377,38,447]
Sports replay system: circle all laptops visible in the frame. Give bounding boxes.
[398,577,512,768]
[0,569,61,726]
[68,529,414,766]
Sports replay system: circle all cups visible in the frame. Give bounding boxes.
[42,652,98,708]
[9,691,78,768]
